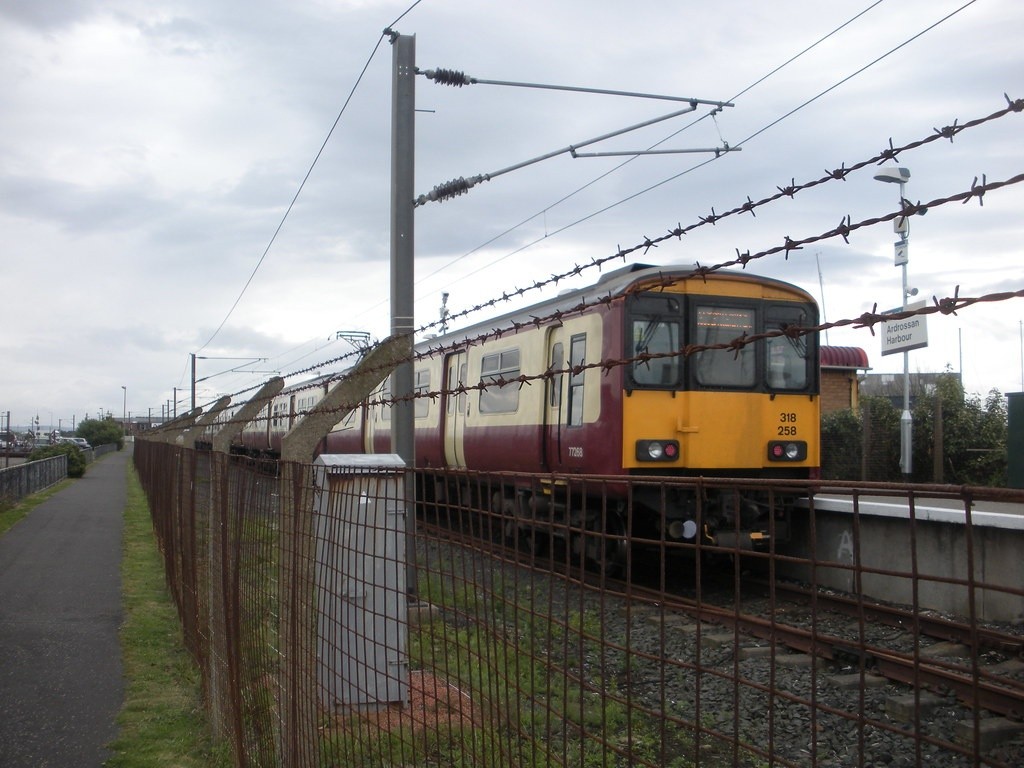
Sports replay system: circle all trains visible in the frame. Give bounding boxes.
[182,263,820,593]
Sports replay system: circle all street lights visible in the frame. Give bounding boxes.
[122,385,127,444]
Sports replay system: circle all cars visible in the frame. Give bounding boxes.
[0,429,90,453]
